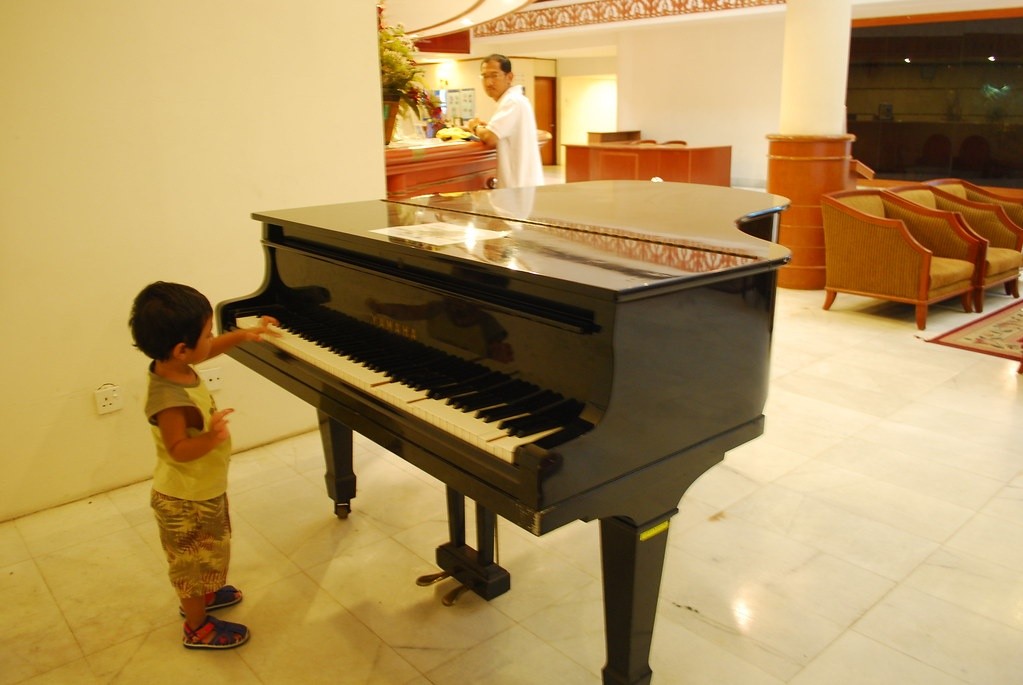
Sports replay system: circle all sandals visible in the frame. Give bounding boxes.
[178,584,243,616]
[182,616,250,650]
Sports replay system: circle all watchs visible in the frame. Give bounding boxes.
[472,123,480,136]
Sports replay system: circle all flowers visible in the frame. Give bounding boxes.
[377,6,460,139]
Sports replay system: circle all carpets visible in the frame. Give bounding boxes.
[928,298,1023,361]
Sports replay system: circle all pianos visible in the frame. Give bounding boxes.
[214,179,793,685]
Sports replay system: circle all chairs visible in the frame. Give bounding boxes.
[819,176,1023,331]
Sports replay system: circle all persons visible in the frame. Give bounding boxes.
[485,189,534,264]
[468,53,545,189]
[130,280,283,649]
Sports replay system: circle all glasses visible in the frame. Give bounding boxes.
[480,72,507,80]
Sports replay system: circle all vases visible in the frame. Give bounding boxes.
[383,100,401,145]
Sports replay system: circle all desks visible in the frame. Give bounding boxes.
[587,131,640,143]
[560,143,732,188]
